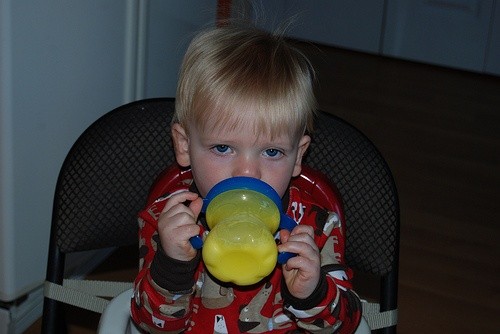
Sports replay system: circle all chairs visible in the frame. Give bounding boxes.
[39,96,402,334]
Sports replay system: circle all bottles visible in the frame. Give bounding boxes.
[184,175,300,286]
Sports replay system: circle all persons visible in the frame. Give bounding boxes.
[131,24,365,333]
